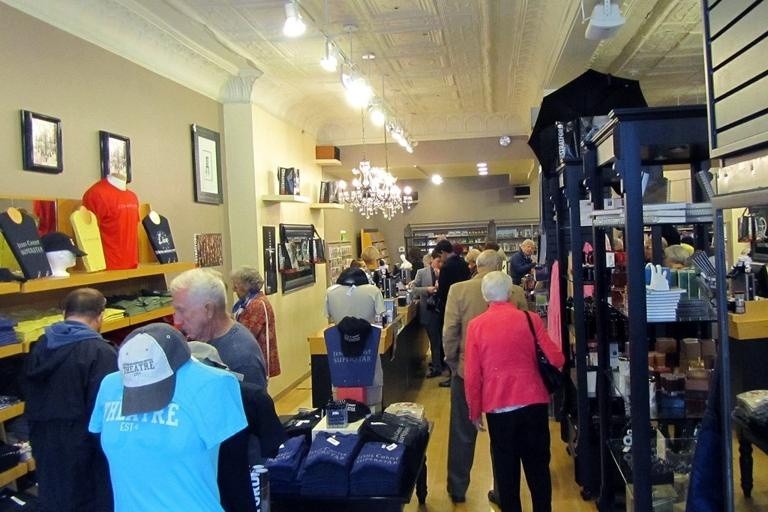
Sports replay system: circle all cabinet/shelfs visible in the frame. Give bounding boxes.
[1,198,196,489]
[404,217,492,256]
[699,0,767,512]
[581,104,718,511]
[493,217,541,259]
[541,115,665,487]
[360,228,391,270]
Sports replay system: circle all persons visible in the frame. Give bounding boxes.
[442,250,529,509]
[231,265,281,378]
[325,239,538,388]
[22,288,117,511]
[738,248,753,274]
[82,172,140,270]
[614,226,696,269]
[463,270,566,512]
[169,268,268,390]
[88,323,257,512]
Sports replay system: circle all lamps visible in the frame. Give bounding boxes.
[380,122,401,219]
[284,0,418,154]
[337,108,393,219]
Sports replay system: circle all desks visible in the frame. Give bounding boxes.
[267,414,435,512]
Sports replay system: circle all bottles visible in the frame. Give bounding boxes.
[733,290,746,314]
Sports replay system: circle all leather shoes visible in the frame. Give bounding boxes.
[426,362,450,387]
[488,490,496,502]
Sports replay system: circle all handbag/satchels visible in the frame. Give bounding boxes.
[537,347,563,393]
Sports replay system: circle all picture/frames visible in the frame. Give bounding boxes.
[191,122,223,206]
[20,109,64,174]
[100,130,132,184]
[279,223,318,296]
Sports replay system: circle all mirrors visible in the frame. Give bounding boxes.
[0,198,58,240]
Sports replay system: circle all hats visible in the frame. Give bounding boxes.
[41,232,88,256]
[337,268,369,286]
[118,322,191,415]
[338,317,371,357]
